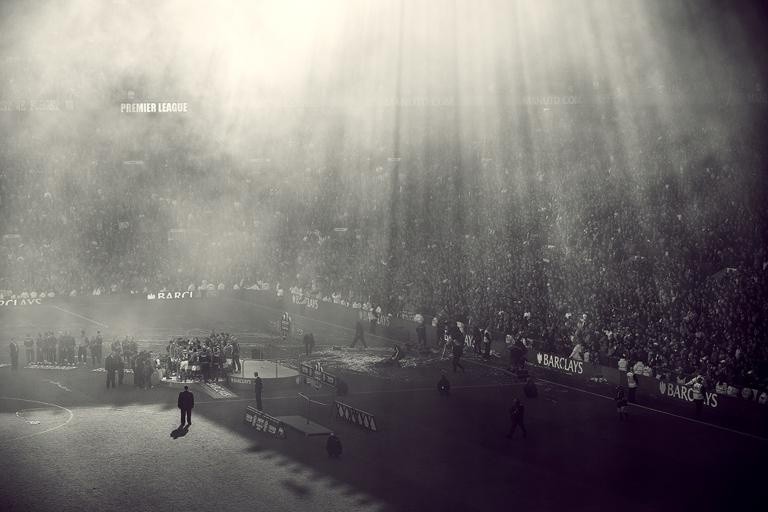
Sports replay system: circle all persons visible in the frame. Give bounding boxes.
[0,0,768,459]
